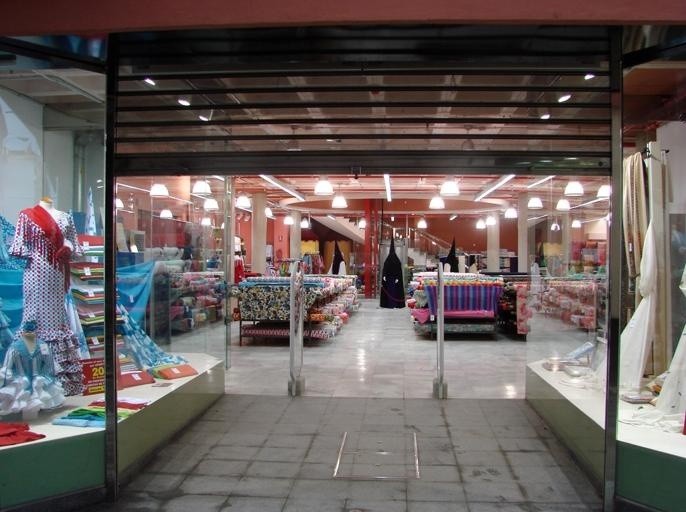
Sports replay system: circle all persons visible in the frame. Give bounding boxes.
[0,197,87,396]
[0,331,66,417]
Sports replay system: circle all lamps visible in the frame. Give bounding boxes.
[416,216,427,228]
[359,213,366,229]
[314,174,334,195]
[115,177,310,229]
[429,188,445,210]
[331,183,348,209]
[439,176,460,197]
[476,176,611,231]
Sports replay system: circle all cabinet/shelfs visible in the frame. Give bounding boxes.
[406,271,530,340]
[237,273,358,347]
